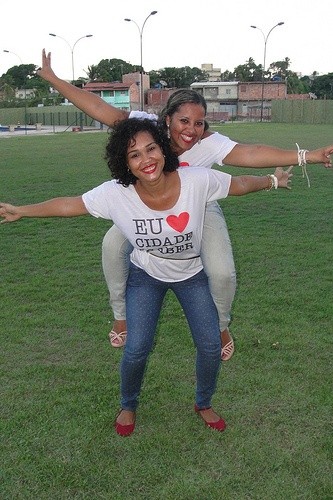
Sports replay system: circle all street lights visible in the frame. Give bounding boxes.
[124,10,158,112]
[3,49,27,135]
[250,22,285,122]
[49,33,93,86]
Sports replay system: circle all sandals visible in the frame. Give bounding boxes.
[220,327,234,360]
[108,325,128,347]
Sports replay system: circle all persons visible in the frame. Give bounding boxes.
[37,48,333,360]
[0,117,294,438]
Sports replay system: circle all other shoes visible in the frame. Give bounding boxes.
[114,408,136,435]
[195,403,225,432]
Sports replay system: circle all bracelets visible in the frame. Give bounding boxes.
[264,174,279,191]
[296,142,310,187]
